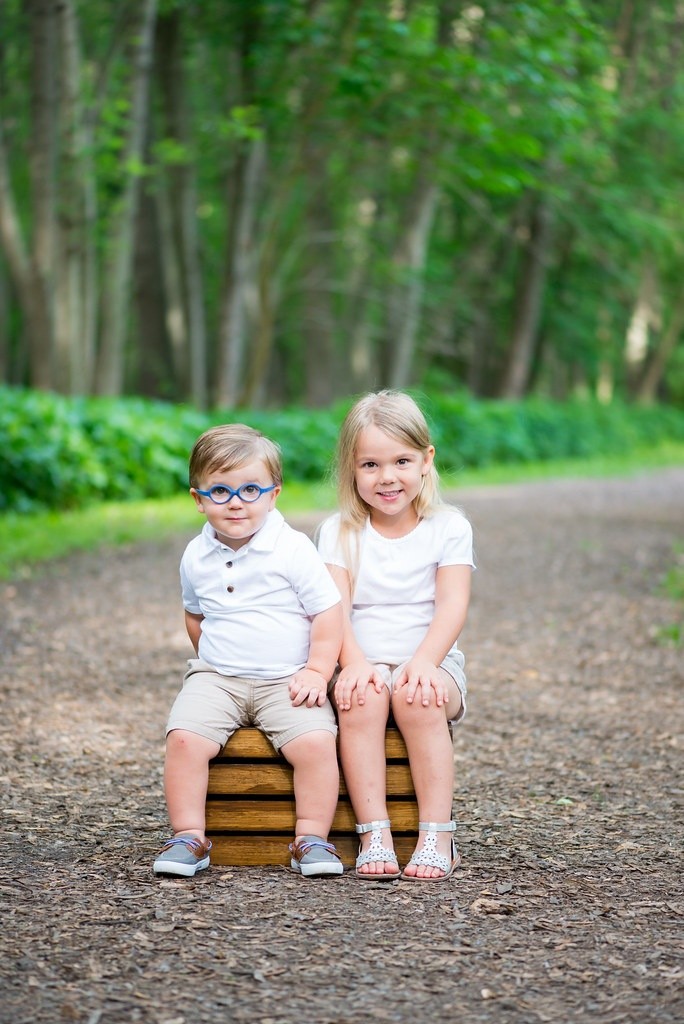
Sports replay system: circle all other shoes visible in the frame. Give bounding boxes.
[153,833,213,877]
[290,834,344,876]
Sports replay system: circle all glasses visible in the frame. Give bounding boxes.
[196,482,277,505]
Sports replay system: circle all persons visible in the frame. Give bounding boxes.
[318,394,477,882]
[153,424,344,876]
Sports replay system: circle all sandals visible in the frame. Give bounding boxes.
[356,819,401,882]
[401,821,462,885]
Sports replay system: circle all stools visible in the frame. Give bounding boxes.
[205,722,454,866]
